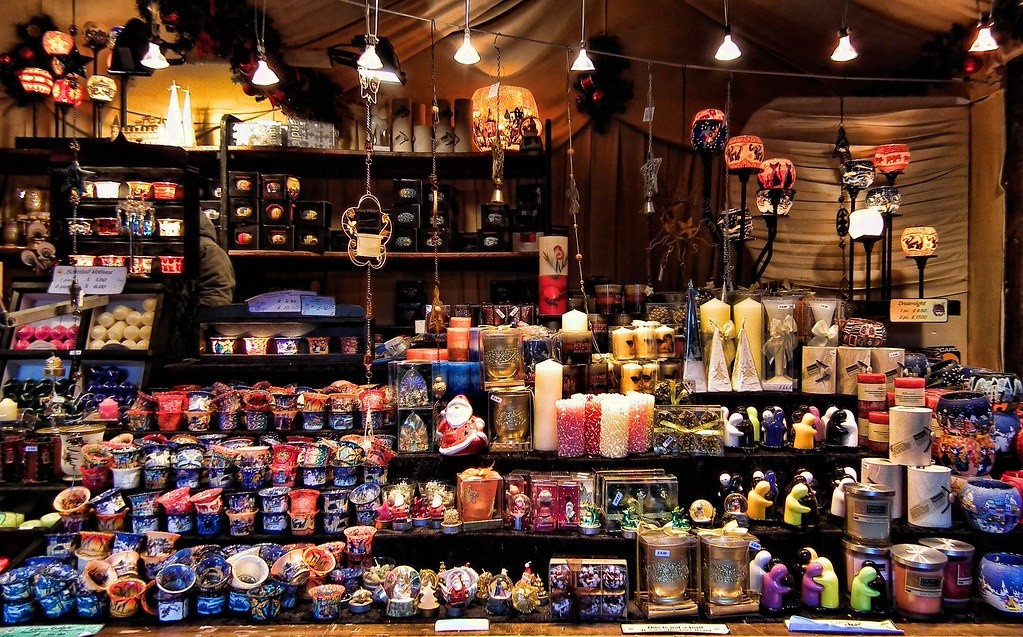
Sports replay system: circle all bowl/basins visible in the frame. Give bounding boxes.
[0,380,397,624]
[67,182,183,273]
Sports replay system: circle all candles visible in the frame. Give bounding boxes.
[368,92,474,153]
[0,298,158,422]
[391,236,976,616]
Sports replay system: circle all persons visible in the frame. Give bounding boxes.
[390,572,411,599]
[167,209,235,360]
[447,572,469,608]
[435,394,488,457]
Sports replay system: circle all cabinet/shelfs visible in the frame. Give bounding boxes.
[0,114,553,344]
[0,137,203,369]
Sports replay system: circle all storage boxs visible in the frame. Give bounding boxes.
[83,294,161,352]
[9,289,87,353]
[227,170,506,251]
[0,351,75,405]
[73,353,153,413]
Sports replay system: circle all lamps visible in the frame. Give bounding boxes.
[714,0,742,61]
[830,0,858,63]
[328,34,404,86]
[18,30,118,140]
[140,0,171,70]
[569,0,596,70]
[968,0,999,53]
[454,0,481,65]
[695,107,937,303]
[251,0,280,86]
[355,0,383,68]
[107,18,184,76]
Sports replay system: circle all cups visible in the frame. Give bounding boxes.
[274,337,301,355]
[210,337,237,353]
[243,338,270,355]
[306,336,331,355]
